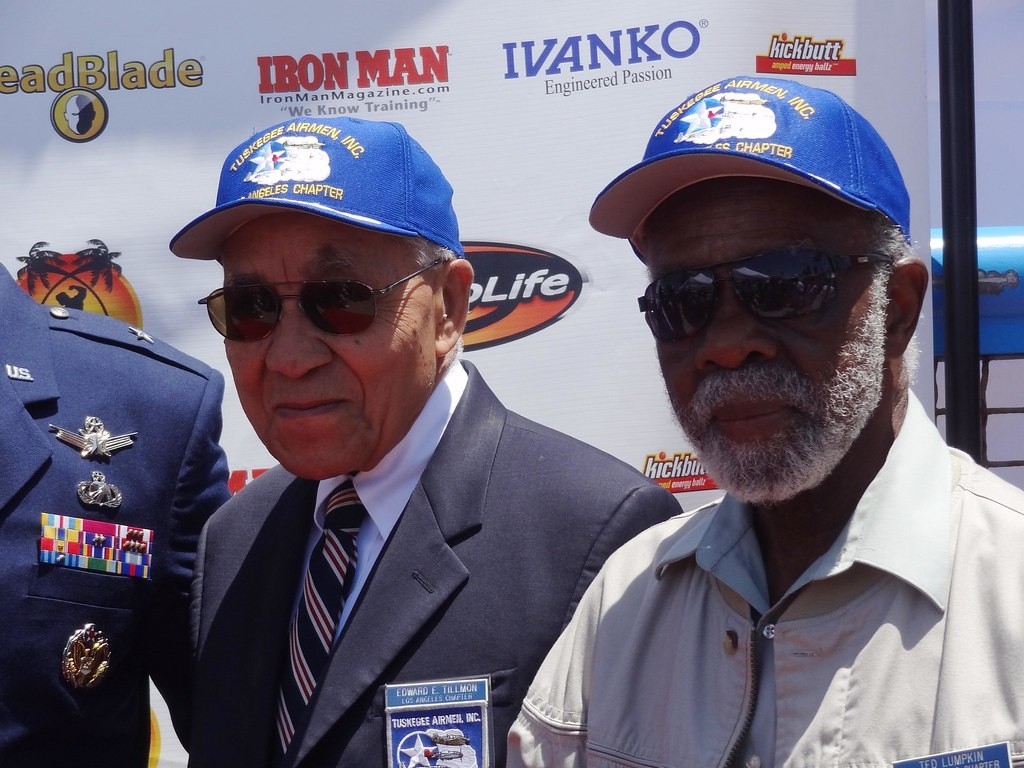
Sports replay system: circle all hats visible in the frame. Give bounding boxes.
[169,116,467,260]
[587,75,912,247]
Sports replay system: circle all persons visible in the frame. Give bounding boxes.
[506,76,1024,768]
[178,115,685,768]
[0,261,233,768]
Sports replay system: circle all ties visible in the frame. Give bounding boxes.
[272,477,368,755]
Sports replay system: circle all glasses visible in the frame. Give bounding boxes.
[197,256,453,342]
[637,248,893,343]
